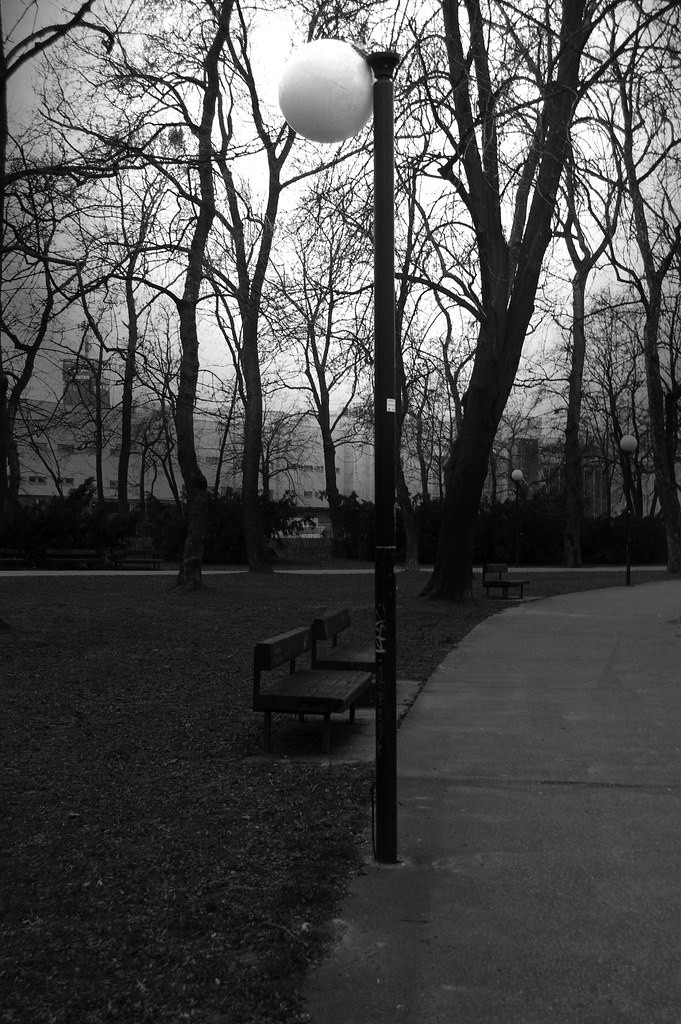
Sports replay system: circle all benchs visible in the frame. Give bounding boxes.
[111,550,165,570]
[0,549,25,570]
[46,550,97,570]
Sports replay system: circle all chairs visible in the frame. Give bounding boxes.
[483,564,530,600]
[253,608,375,755]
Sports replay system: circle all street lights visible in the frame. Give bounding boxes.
[620,435,637,585]
[283,34,400,861]
[511,469,524,566]
